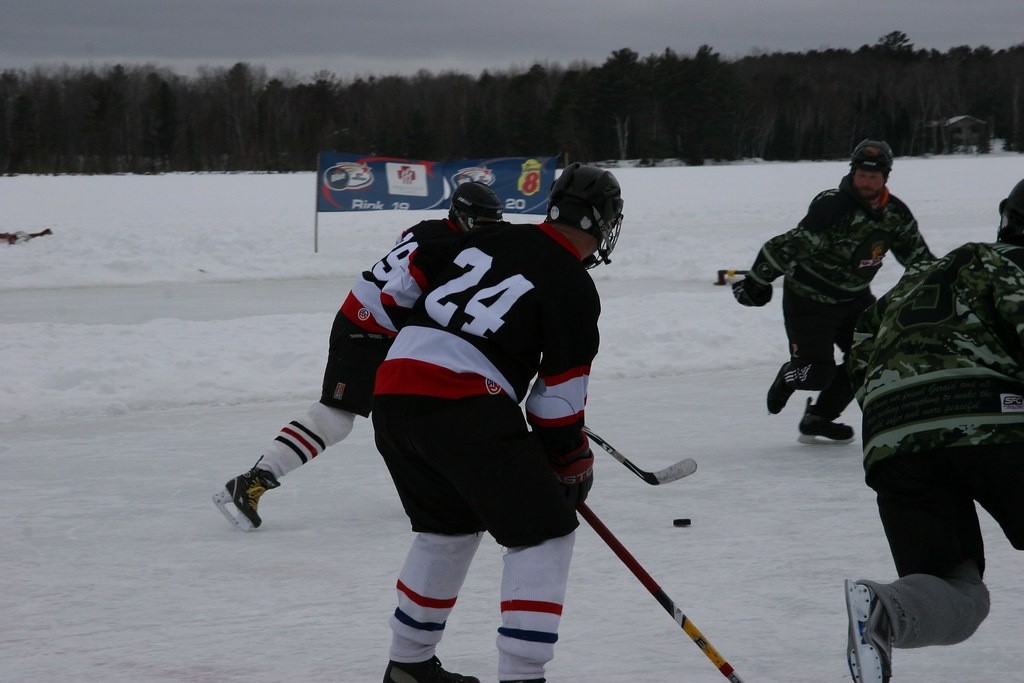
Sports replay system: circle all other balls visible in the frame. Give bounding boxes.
[673,519,691,528]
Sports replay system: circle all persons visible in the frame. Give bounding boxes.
[213,183,502,532]
[733,138,938,445]
[846,176,1024,683]
[372,163,623,683]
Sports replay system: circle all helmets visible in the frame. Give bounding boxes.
[449,181,502,221]
[1000,180,1023,215]
[546,162,623,236]
[850,139,893,172]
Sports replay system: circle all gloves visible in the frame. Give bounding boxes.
[731,277,772,305]
[532,417,595,514]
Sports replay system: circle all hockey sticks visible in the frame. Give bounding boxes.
[575,502,744,683]
[583,424,698,487]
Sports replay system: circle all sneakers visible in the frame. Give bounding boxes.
[798,397,855,446]
[213,466,281,531]
[845,579,893,683]
[383,655,479,683]
[767,361,795,414]
